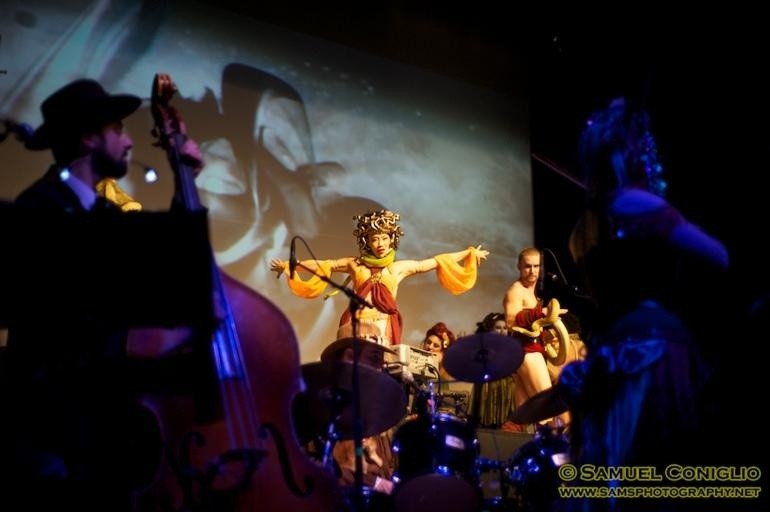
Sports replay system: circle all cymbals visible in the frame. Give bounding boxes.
[442,334,526,385]
[296,360,409,441]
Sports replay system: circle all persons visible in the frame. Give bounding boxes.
[1,80,205,510]
[270,117,730,508]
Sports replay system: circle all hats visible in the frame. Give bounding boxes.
[25,79,141,150]
[425,323,455,350]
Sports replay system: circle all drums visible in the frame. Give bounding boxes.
[386,412,482,512]
[506,437,574,509]
[331,483,391,512]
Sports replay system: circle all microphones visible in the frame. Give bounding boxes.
[401,370,421,394]
[539,253,545,291]
[289,239,296,280]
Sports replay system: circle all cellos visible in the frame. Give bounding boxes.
[120,72,344,512]
